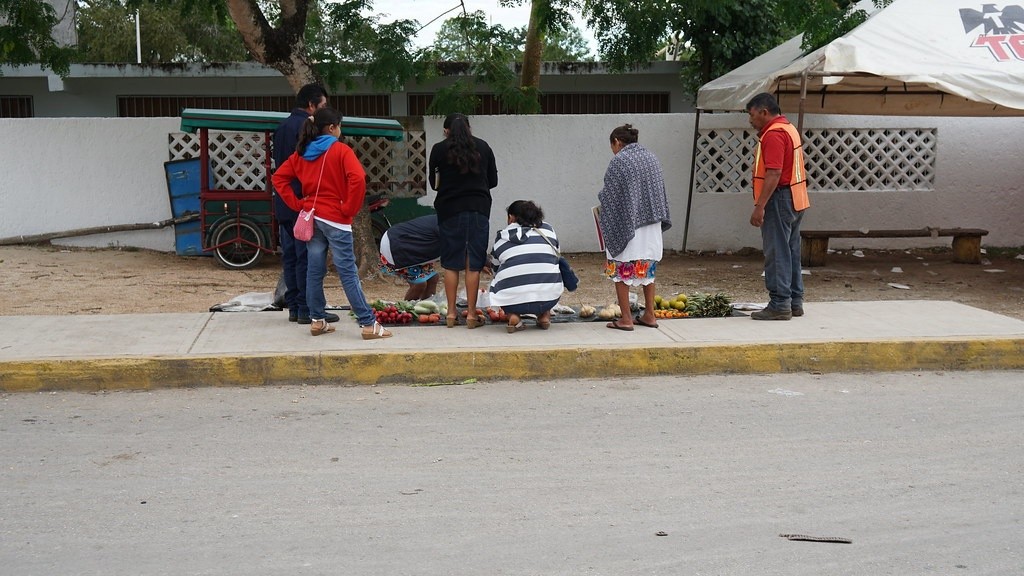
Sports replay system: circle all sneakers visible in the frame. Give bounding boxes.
[791,304,804,316]
[751,307,791,320]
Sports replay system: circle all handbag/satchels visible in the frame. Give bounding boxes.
[559,256,579,292]
[293,208,315,241]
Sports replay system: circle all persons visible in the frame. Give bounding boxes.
[598,124,671,331]
[380,214,441,301]
[271,107,393,339]
[429,114,498,329]
[746,93,811,320]
[273,84,340,323]
[484,199,563,333]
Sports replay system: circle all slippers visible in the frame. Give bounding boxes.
[632,316,658,327]
[606,320,634,331]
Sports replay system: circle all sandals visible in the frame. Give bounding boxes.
[310,318,335,336]
[361,321,393,339]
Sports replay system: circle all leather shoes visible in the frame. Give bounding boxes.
[298,311,339,324]
[289,310,298,322]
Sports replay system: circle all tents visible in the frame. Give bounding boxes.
[681,0,1023,254]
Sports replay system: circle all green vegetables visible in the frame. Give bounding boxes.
[684,294,733,318]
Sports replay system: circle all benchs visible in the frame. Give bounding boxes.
[799,229,989,268]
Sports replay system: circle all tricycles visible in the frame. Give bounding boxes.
[180,109,406,270]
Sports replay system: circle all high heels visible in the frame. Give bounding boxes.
[536,314,550,330]
[466,315,486,329]
[446,311,459,328]
[506,319,526,333]
